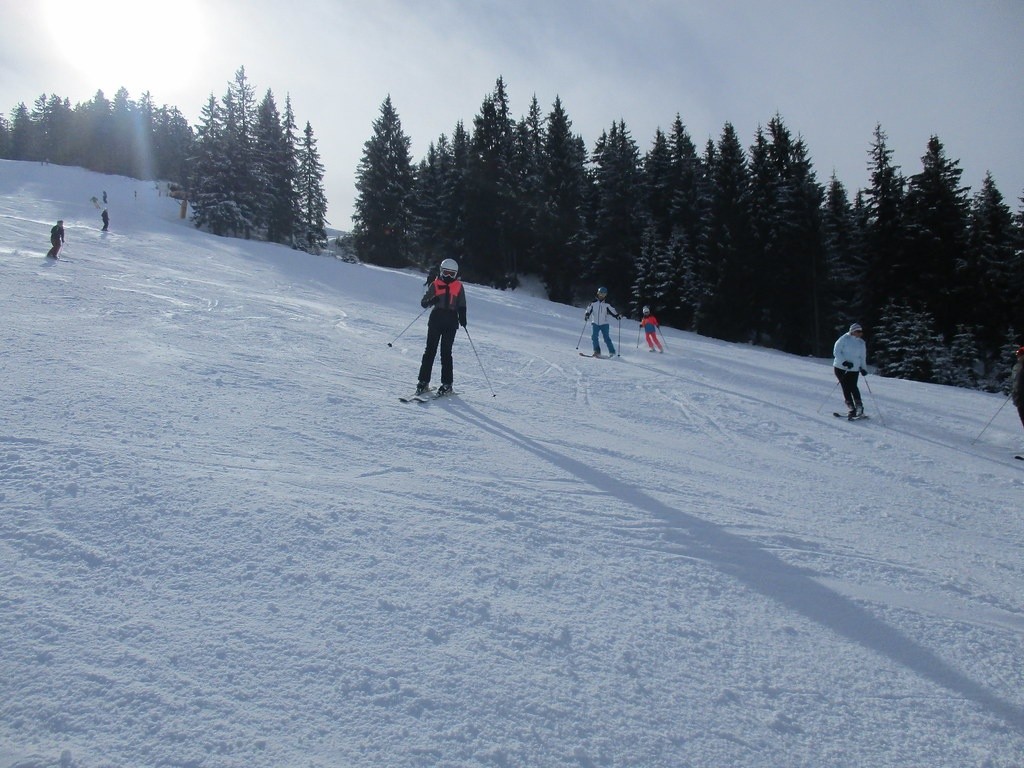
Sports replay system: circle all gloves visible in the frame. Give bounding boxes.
[861,370,867,376]
[459,318,467,327]
[842,361,853,369]
[427,296,439,305]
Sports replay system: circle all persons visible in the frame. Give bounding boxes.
[90,196,103,208]
[47,219,64,260]
[102,189,108,204]
[100,207,109,231]
[1003,346,1024,459]
[638,306,665,354]
[414,259,468,399]
[422,260,440,288]
[832,323,868,419]
[584,286,624,355]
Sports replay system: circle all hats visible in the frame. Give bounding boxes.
[849,323,863,333]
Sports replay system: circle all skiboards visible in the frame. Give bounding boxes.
[398,385,463,402]
[579,352,618,359]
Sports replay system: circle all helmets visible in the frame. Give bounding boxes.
[598,287,607,293]
[440,258,458,279]
[643,308,650,313]
[1015,346,1024,357]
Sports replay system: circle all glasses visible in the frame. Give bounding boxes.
[853,330,862,333]
[441,269,457,278]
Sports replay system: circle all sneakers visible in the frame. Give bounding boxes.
[853,400,863,416]
[846,400,855,416]
[437,385,452,395]
[416,382,429,395]
